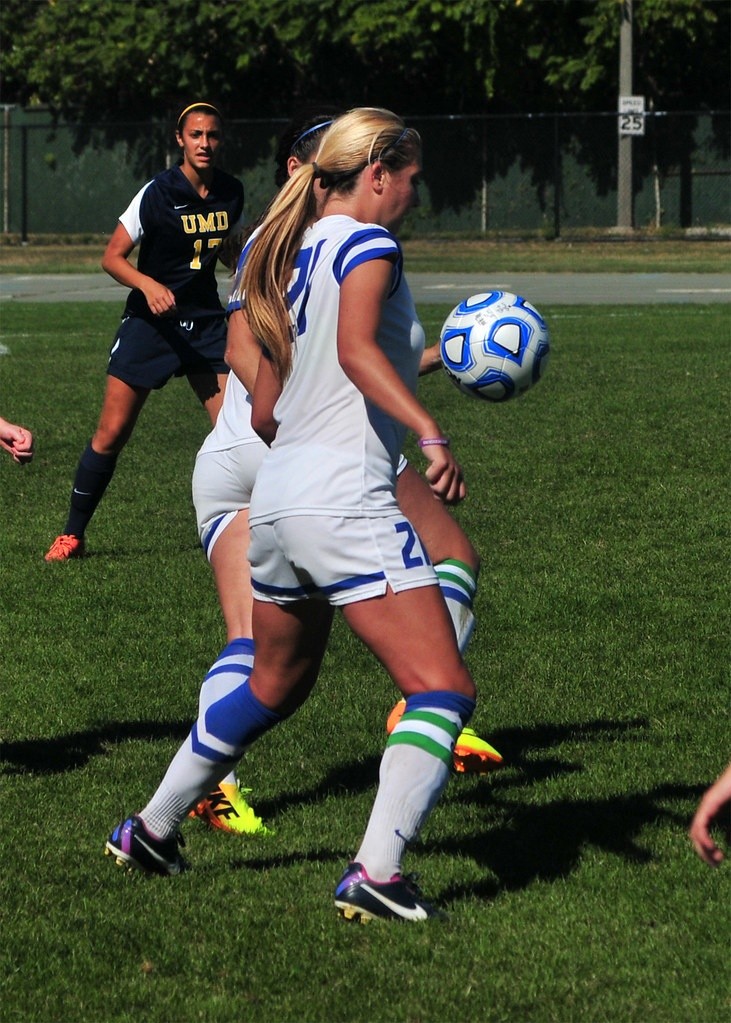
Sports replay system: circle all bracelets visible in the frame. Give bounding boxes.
[419,438,450,448]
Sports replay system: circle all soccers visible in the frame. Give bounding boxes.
[438,289,552,404]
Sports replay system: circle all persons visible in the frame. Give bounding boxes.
[102,108,505,926]
[45,103,246,561]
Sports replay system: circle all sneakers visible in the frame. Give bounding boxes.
[187,778,274,839]
[44,534,83,562]
[333,861,447,927]
[386,696,504,774]
[105,812,191,878]
